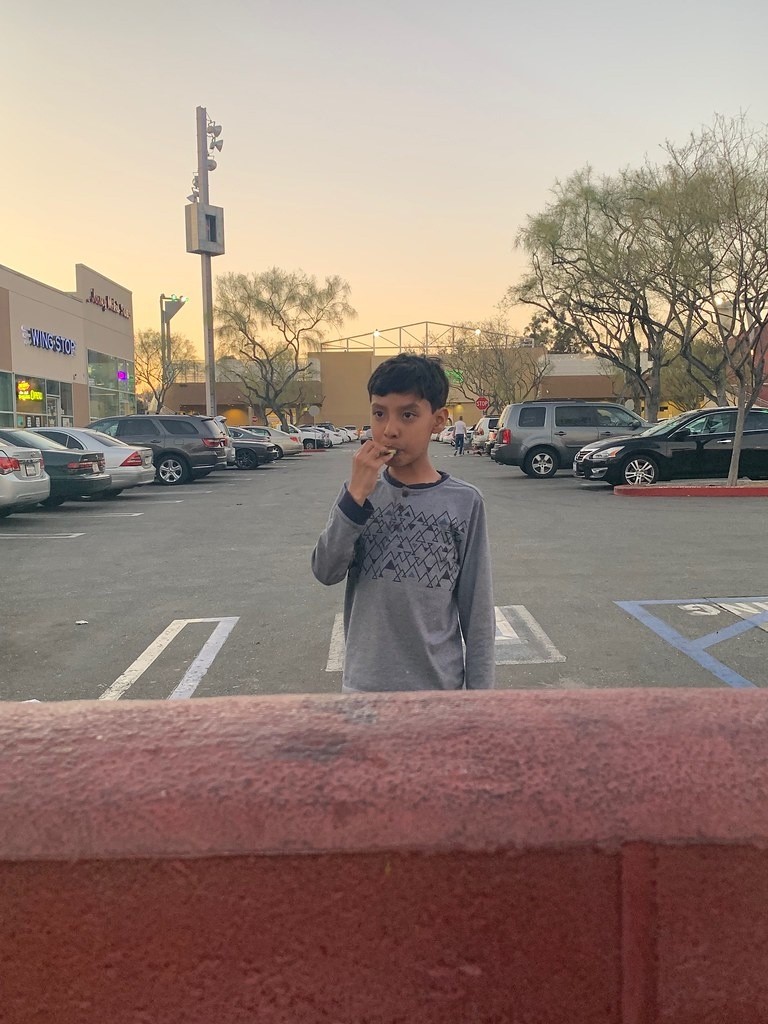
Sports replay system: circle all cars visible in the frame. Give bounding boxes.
[240,426,303,460]
[0,427,112,507]
[570,405,768,487]
[430,424,475,448]
[225,427,277,471]
[278,422,360,450]
[26,425,157,501]
[0,438,51,518]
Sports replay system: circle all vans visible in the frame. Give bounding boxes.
[214,415,236,467]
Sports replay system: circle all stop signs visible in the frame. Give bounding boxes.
[476,397,490,410]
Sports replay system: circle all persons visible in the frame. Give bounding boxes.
[452,416,468,457]
[310,352,495,694]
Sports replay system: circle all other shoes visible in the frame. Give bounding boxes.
[454,450,458,456]
[459,454,463,456]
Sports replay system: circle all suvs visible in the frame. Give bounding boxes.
[470,413,503,449]
[360,425,373,444]
[488,398,656,480]
[88,414,228,486]
